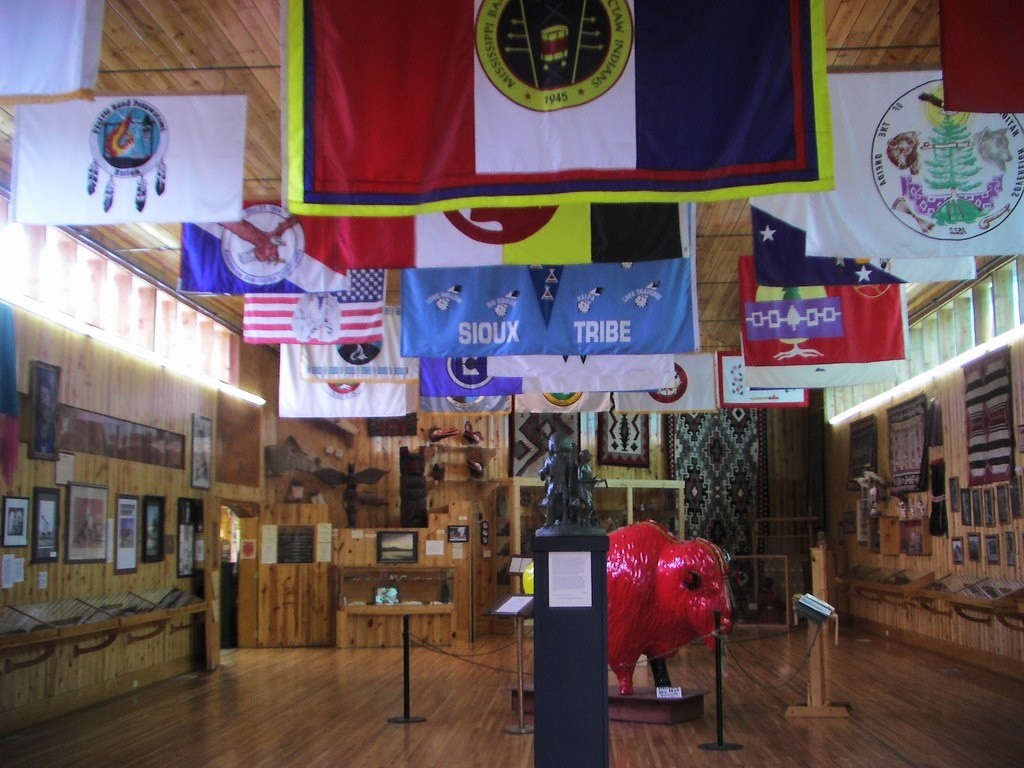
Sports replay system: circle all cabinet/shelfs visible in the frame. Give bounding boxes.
[421,441,497,486]
[491,476,685,631]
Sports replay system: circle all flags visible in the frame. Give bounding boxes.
[0,1,1024,418]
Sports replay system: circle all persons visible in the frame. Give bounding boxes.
[539,432,609,529]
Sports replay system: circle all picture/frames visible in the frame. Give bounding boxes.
[984,533,1001,566]
[176,497,205,579]
[143,494,165,564]
[1004,531,1017,567]
[112,493,140,574]
[1,495,30,548]
[950,535,965,564]
[971,488,984,527]
[65,481,109,566]
[32,486,58,564]
[966,532,982,563]
[1009,475,1024,518]
[28,359,62,460]
[948,476,960,513]
[448,525,469,543]
[996,483,1012,526]
[189,412,213,491]
[960,488,972,526]
[984,487,997,528]
[377,530,418,565]
